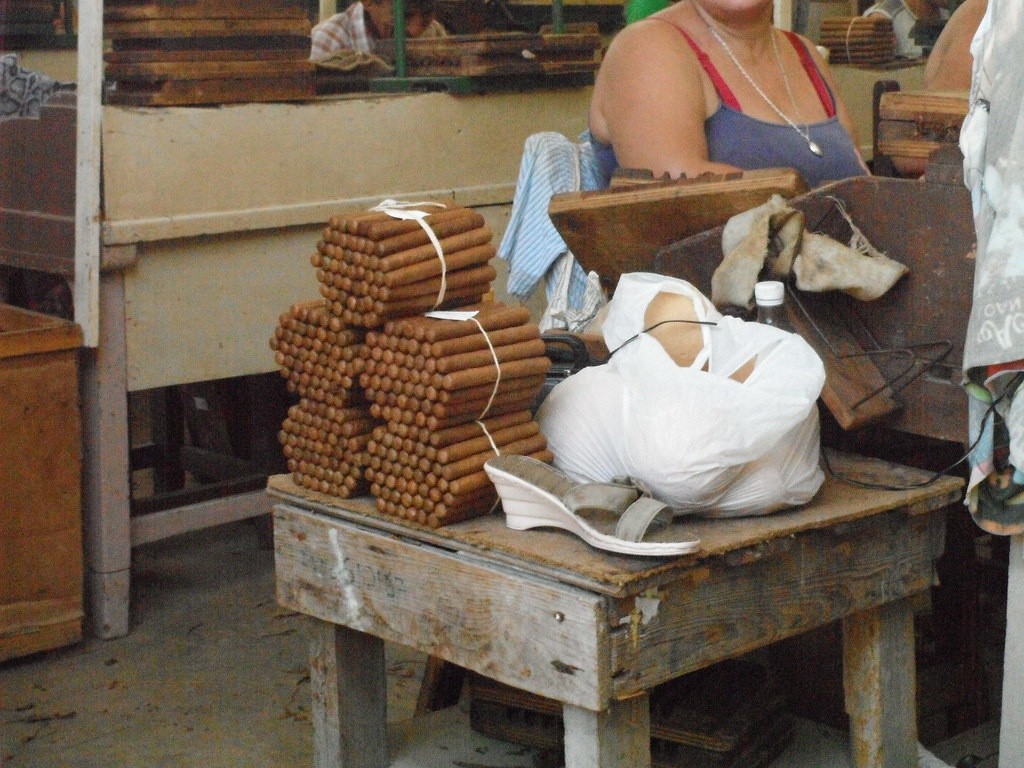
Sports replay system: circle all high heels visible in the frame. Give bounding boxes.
[484,456,700,558]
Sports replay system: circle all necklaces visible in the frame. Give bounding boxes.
[712,22,823,157]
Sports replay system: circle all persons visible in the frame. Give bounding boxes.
[588,0,872,191]
[863,0,952,58]
[310,0,448,65]
[923,0,988,88]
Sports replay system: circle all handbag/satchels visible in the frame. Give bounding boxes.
[526,270,830,518]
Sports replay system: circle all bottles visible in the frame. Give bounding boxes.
[754,281,790,332]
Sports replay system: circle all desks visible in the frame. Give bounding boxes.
[265,451,964,768]
